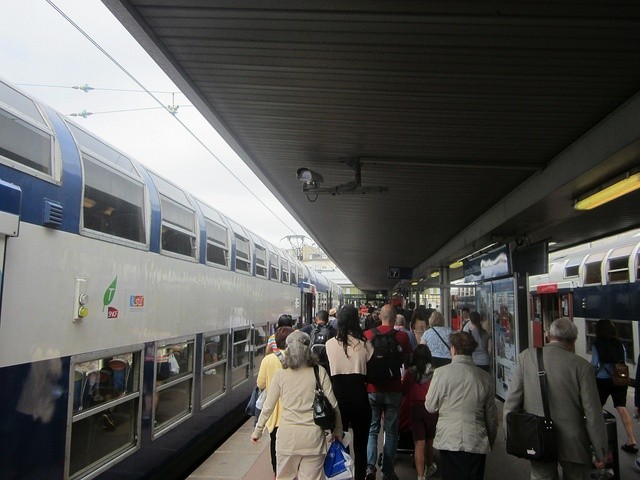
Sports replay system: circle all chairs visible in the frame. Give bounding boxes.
[107,358,129,395]
[73,368,86,428]
[85,371,110,407]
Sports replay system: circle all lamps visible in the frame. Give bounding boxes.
[573,172,640,210]
[449,260,463,269]
[411,282,418,285]
[430,271,439,278]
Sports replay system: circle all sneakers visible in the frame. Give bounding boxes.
[382,471,399,480]
[424,462,438,476]
[364,467,376,479]
[101,413,117,427]
[621,442,637,453]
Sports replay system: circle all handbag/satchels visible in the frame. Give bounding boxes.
[312,364,336,430]
[244,386,258,416]
[158,360,170,381]
[505,346,560,463]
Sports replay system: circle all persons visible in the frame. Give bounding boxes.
[256,326,295,477]
[409,308,426,344]
[458,307,470,332]
[405,302,416,321]
[96,353,133,430]
[426,304,434,312]
[86,361,104,403]
[263,313,293,354]
[359,302,381,327]
[14,332,62,467]
[142,343,180,426]
[452,308,460,332]
[203,334,220,376]
[469,311,492,372]
[420,311,453,365]
[299,309,335,373]
[362,303,412,480]
[628,351,640,469]
[589,316,636,452]
[245,325,257,378]
[499,301,513,324]
[327,307,337,329]
[418,304,430,329]
[504,317,609,479]
[423,330,499,480]
[397,344,441,480]
[394,314,416,349]
[492,310,500,320]
[396,304,405,314]
[325,304,374,480]
[436,305,440,312]
[251,329,343,480]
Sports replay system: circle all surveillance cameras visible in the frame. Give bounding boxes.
[296,168,323,189]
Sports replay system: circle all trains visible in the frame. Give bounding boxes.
[0,74,345,480]
[463,229,640,384]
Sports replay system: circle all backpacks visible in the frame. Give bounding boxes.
[365,327,399,385]
[309,324,333,362]
[600,363,630,387]
